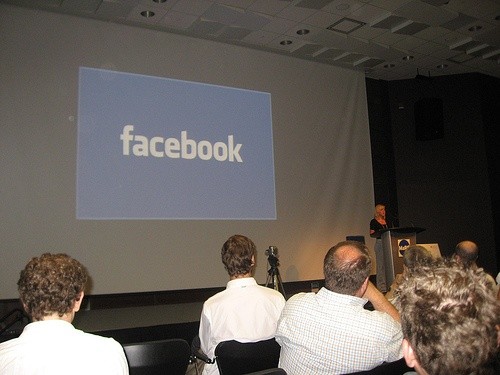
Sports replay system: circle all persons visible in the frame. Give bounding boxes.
[274,241,403,375]
[369,205,396,293]
[185,235,286,375]
[386,240,500,375]
[0,252,129,375]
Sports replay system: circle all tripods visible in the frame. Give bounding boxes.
[265,257,286,299]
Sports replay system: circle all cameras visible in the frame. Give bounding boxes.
[265,246,278,257]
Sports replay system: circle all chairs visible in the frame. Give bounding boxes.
[191,335,281,375]
[122,339,199,375]
